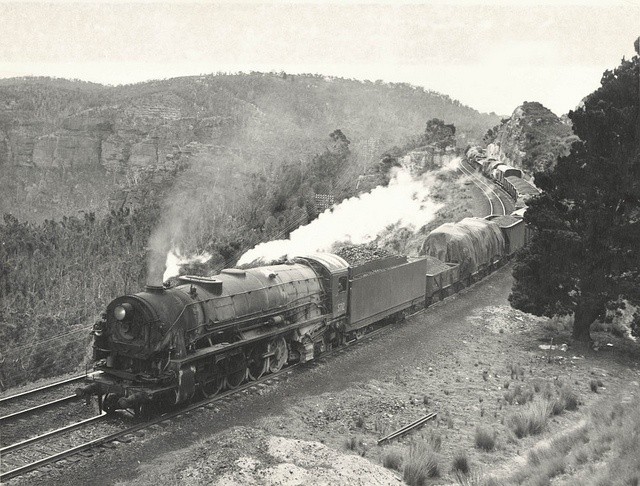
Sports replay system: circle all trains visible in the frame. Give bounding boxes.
[75,146,541,424]
